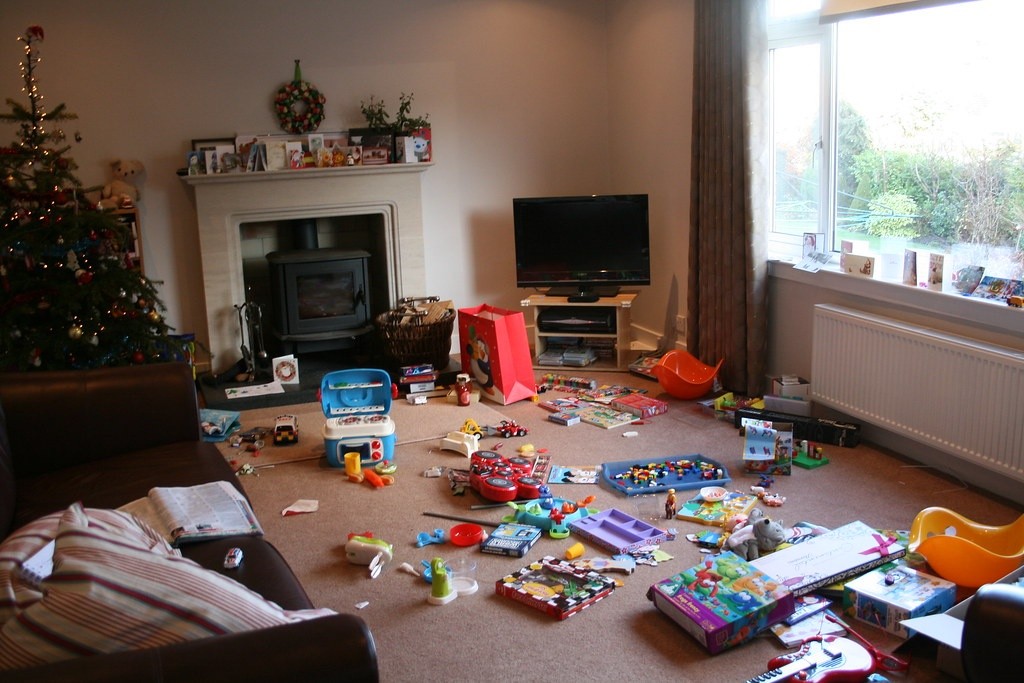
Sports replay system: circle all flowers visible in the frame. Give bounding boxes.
[275,79,326,135]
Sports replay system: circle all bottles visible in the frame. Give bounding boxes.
[455,372,472,406]
[802,440,822,460]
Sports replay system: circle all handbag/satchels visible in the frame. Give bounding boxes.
[458,303,537,406]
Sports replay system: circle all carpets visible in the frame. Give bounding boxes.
[210,390,514,471]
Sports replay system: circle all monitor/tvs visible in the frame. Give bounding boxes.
[513,194,650,297]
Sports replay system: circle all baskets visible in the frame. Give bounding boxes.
[377,308,455,370]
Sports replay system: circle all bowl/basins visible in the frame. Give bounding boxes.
[650,351,724,398]
[450,523,482,546]
[908,507,1024,587]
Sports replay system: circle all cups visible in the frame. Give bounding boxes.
[566,542,584,559]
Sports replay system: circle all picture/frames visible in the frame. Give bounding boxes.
[346,127,396,163]
[308,134,324,153]
[190,137,236,160]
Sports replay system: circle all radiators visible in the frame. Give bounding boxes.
[808,302,1024,485]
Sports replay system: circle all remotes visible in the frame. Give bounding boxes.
[568,297,599,302]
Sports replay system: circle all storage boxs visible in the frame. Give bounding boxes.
[495,556,615,621]
[898,565,1024,683]
[646,551,795,654]
[842,562,957,638]
[480,523,541,557]
[611,392,669,418]
[569,508,669,556]
[397,136,415,163]
[399,364,439,393]
[747,521,906,595]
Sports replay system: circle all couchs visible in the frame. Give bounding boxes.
[0,361,381,683]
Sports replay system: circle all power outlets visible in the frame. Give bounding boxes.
[675,316,685,333]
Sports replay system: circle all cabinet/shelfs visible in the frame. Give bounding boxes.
[521,294,638,372]
[102,207,144,277]
